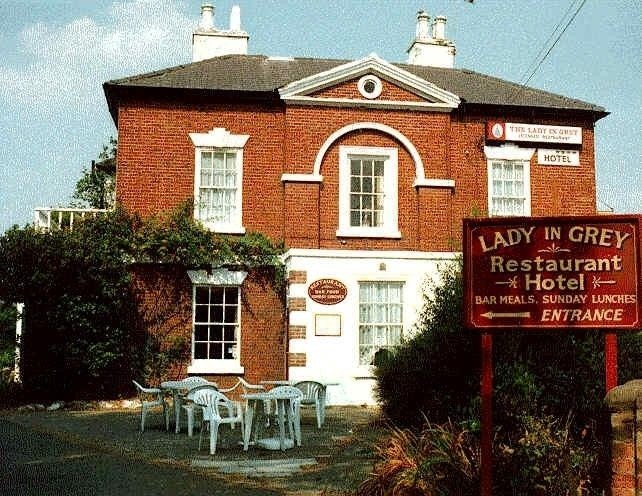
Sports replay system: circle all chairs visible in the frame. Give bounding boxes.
[130,370,327,457]
